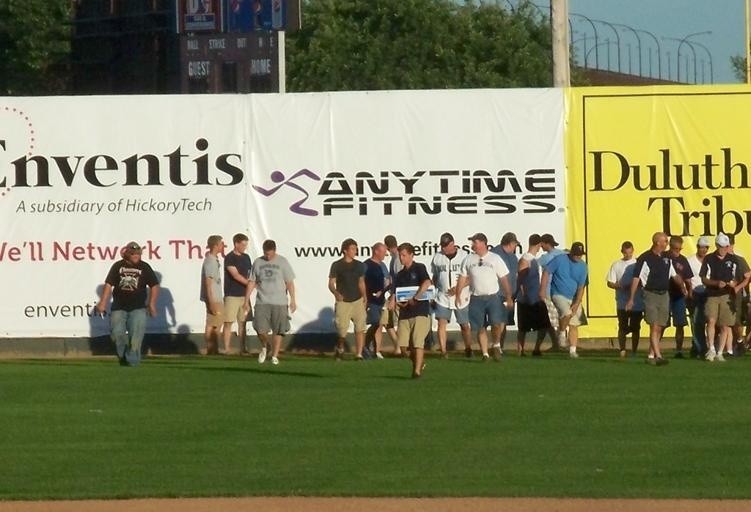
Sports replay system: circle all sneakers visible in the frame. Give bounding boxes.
[240,351,249,356]
[420,363,426,372]
[531,350,543,357]
[716,350,725,362]
[518,352,526,358]
[334,345,345,363]
[489,345,504,361]
[481,355,491,363]
[655,356,670,367]
[723,351,735,360]
[464,348,474,359]
[704,349,717,362]
[412,370,421,378]
[558,333,568,348]
[223,350,237,357]
[257,347,267,364]
[569,350,579,361]
[645,356,655,366]
[674,351,686,360]
[736,340,747,353]
[439,351,449,360]
[271,356,279,365]
[374,351,384,360]
[363,347,371,360]
[354,354,364,363]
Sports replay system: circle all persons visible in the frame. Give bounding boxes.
[328,232,589,377]
[241,239,297,365]
[96,241,160,368]
[604,231,750,363]
[199,235,228,354]
[222,233,253,357]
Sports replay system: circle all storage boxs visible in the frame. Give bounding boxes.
[395,285,435,302]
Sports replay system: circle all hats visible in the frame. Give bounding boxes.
[715,234,730,248]
[127,242,142,254]
[500,232,519,245]
[698,237,711,247]
[438,233,454,246]
[570,242,586,257]
[468,233,487,244]
[540,233,559,247]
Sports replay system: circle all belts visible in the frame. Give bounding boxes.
[644,287,668,295]
